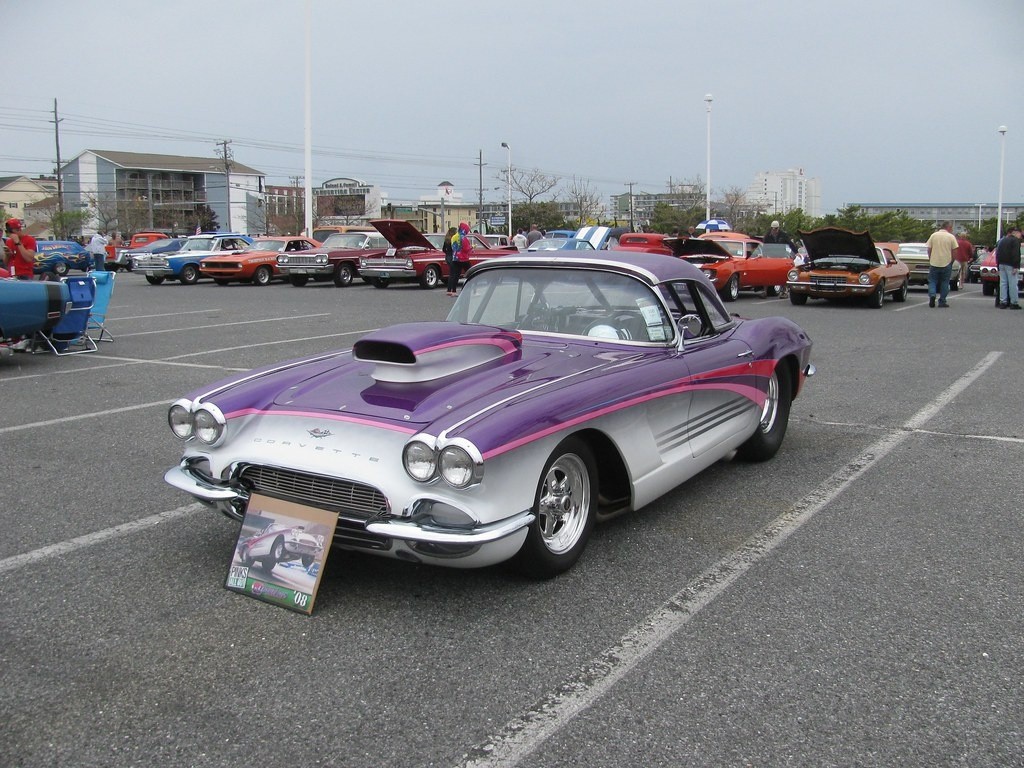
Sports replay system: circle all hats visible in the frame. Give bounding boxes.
[771,221,779,227]
[6,219,22,228]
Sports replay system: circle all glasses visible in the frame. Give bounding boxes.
[5,230,14,234]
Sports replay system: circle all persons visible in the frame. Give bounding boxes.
[928,221,991,307]
[0,218,38,351]
[512,223,543,250]
[92,230,125,271]
[762,220,809,297]
[671,225,698,238]
[442,223,478,297]
[996,227,1023,309]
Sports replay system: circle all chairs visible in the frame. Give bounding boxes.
[31,277,99,356]
[70,271,116,344]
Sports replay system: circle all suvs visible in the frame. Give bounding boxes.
[33,240,92,278]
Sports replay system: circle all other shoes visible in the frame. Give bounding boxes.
[473,293,480,297]
[447,292,452,296]
[1000,303,1008,309]
[1010,303,1022,310]
[929,296,936,308]
[938,299,950,307]
[780,292,788,299]
[10,340,32,352]
[451,292,458,297]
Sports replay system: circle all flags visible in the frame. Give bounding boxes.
[195,222,202,235]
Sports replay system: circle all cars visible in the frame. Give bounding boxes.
[359,219,520,289]
[697,231,755,254]
[0,280,72,341]
[161,252,816,575]
[116,237,185,268]
[275,230,401,287]
[236,524,317,572]
[200,236,325,288]
[964,243,1024,295]
[894,240,963,291]
[100,232,175,272]
[661,235,796,301]
[790,225,910,308]
[529,224,661,253]
[130,236,246,285]
[312,224,377,247]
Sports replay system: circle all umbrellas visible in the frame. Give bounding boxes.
[695,218,734,230]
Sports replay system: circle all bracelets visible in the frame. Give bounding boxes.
[15,242,22,245]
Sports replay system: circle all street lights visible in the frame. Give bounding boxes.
[705,93,713,235]
[501,144,512,243]
[996,124,1008,247]
